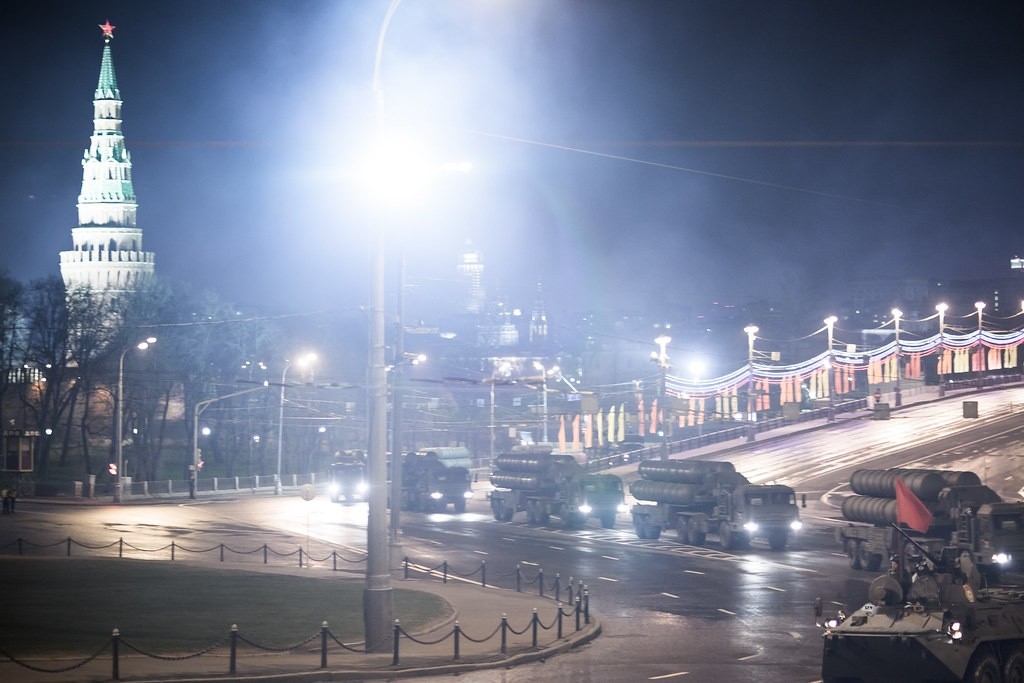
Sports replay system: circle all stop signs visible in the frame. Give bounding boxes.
[874,391,881,400]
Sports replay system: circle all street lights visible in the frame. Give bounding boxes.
[275,352,319,495]
[325,70,465,649]
[744,322,758,443]
[655,333,672,461]
[490,362,512,471]
[892,309,904,405]
[935,303,949,398]
[823,314,838,421]
[109,336,159,502]
[975,301,986,389]
[533,359,560,442]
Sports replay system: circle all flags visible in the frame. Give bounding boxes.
[894,474,934,535]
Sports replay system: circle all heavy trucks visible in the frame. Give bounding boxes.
[487,442,626,528]
[628,460,807,553]
[327,449,370,503]
[836,467,1024,586]
[386,446,477,514]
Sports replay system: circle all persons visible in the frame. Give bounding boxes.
[887,553,912,603]
[1,483,18,514]
[187,465,196,499]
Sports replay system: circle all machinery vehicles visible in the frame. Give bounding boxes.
[812,521,1024,682]
